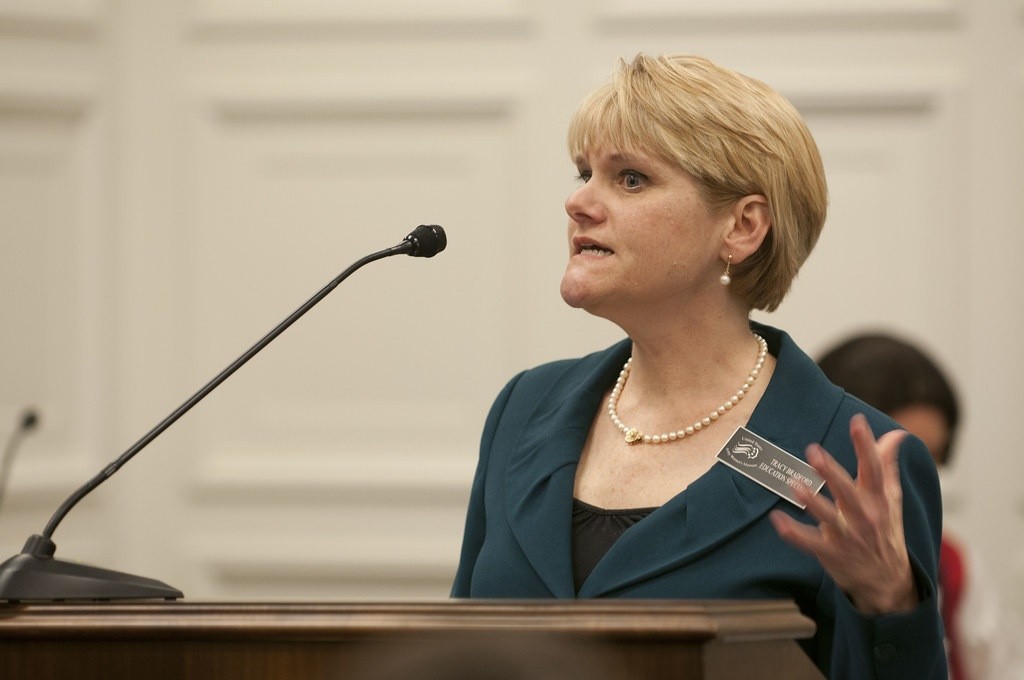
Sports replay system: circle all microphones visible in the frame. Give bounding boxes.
[0,225,448,602]
[0,413,36,492]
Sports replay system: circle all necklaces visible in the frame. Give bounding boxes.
[609,332,767,446]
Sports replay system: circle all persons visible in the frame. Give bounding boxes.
[817,332,1006,680]
[450,52,948,680]
